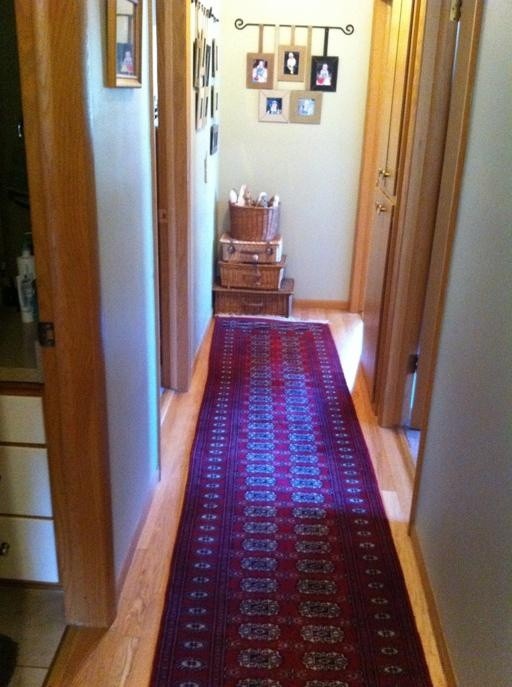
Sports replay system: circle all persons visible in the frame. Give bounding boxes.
[300,99,310,115]
[251,58,268,83]
[316,62,331,84]
[120,48,134,76]
[283,51,297,74]
[266,99,281,114]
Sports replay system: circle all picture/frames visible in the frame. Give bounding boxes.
[213,186,296,317]
[228,19,356,128]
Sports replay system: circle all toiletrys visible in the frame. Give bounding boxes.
[16,239,35,278]
[15,273,35,324]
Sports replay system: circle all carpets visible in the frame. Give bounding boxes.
[147,312,435,683]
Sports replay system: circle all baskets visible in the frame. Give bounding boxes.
[211,275,295,319]
[218,231,283,264]
[217,254,289,291]
[228,198,282,242]
